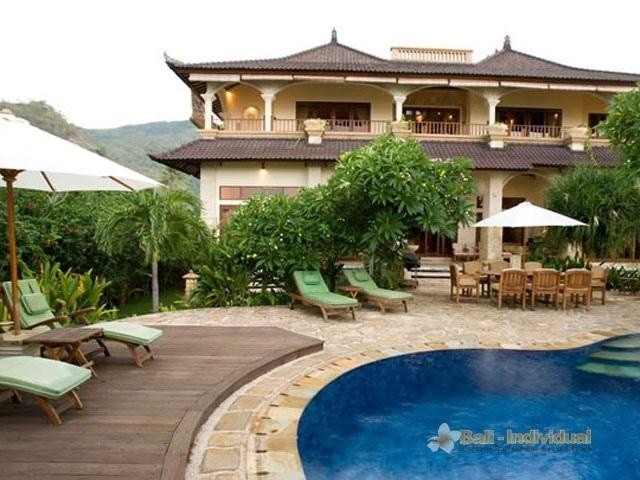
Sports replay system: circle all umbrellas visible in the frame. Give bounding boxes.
[0,108,169,341]
[471,201,591,269]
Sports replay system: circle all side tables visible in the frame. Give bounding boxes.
[22,328,110,379]
[334,286,362,313]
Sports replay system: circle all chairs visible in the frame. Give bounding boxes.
[0,278,164,368]
[339,260,414,315]
[288,270,359,321]
[0,355,92,425]
[450,261,608,311]
